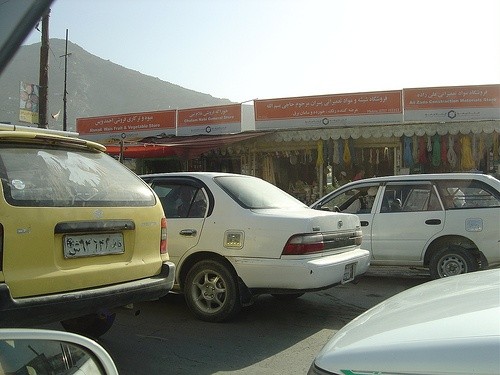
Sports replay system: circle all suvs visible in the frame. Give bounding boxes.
[0,123,177,340]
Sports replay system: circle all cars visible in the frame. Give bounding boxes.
[137,172,373,322]
[306,268,500,375]
[309,172,500,282]
